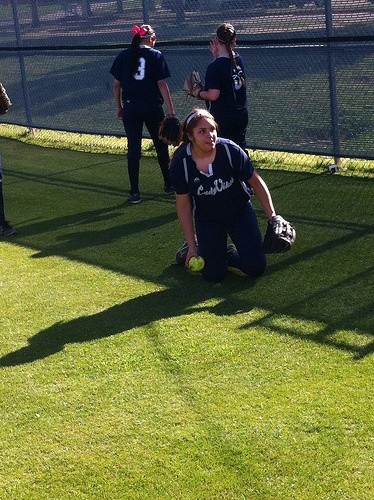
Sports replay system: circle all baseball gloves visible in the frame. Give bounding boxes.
[157,115,183,145]
[183,69,203,101]
[263,216,296,253]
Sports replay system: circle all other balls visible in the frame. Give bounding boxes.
[187,256,205,271]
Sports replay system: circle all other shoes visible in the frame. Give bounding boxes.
[164,186,175,194]
[0,221,16,236]
[226,244,236,269]
[176,234,199,265]
[129,192,141,203]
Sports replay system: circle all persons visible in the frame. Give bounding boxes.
[167,108,299,281]
[0,82,17,237]
[183,23,249,155]
[109,25,176,203]
